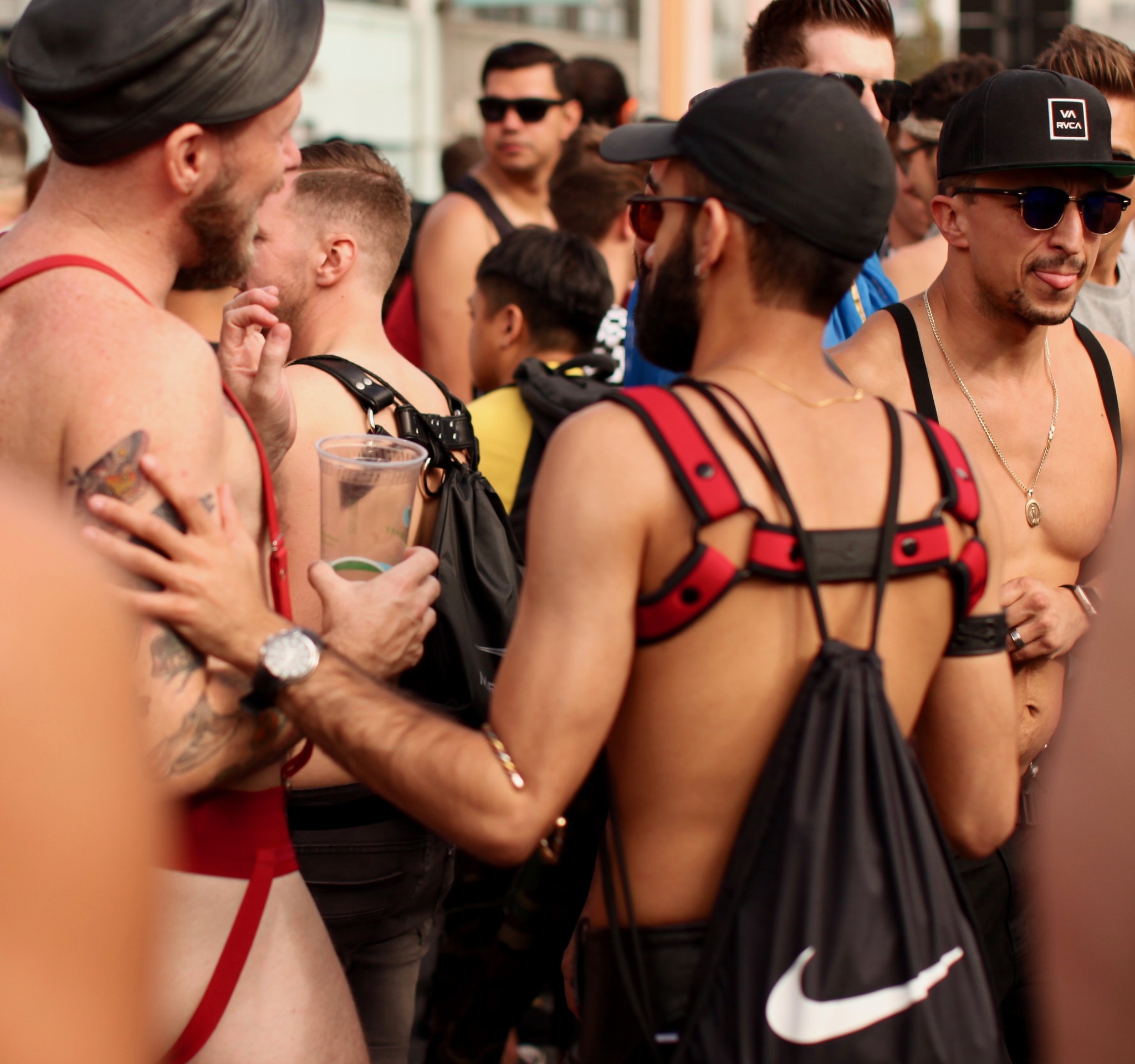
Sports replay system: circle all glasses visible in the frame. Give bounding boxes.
[951,182,1130,238]
[624,190,769,245]
[822,71,918,121]
[894,142,932,174]
[475,92,566,127]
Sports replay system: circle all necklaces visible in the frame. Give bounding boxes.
[923,290,1058,526]
[718,365,861,409]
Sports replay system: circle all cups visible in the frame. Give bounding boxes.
[313,434,429,581]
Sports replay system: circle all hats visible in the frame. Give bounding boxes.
[12,0,332,167]
[938,63,1134,201]
[600,67,898,272]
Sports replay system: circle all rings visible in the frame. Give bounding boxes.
[1010,628,1025,648]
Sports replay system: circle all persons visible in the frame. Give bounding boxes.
[884,123,943,257]
[549,139,650,385]
[81,69,1020,1064]
[1029,447,1135,1064]
[1020,22,1135,355]
[879,54,1006,304]
[441,130,482,190]
[0,0,479,1064]
[623,0,898,387]
[565,57,639,127]
[418,225,613,1064]
[415,41,581,404]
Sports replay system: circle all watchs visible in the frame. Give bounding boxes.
[237,627,326,710]
[1059,585,1104,622]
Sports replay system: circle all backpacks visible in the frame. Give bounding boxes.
[506,349,620,544]
[291,350,522,728]
[679,374,1000,1063]
